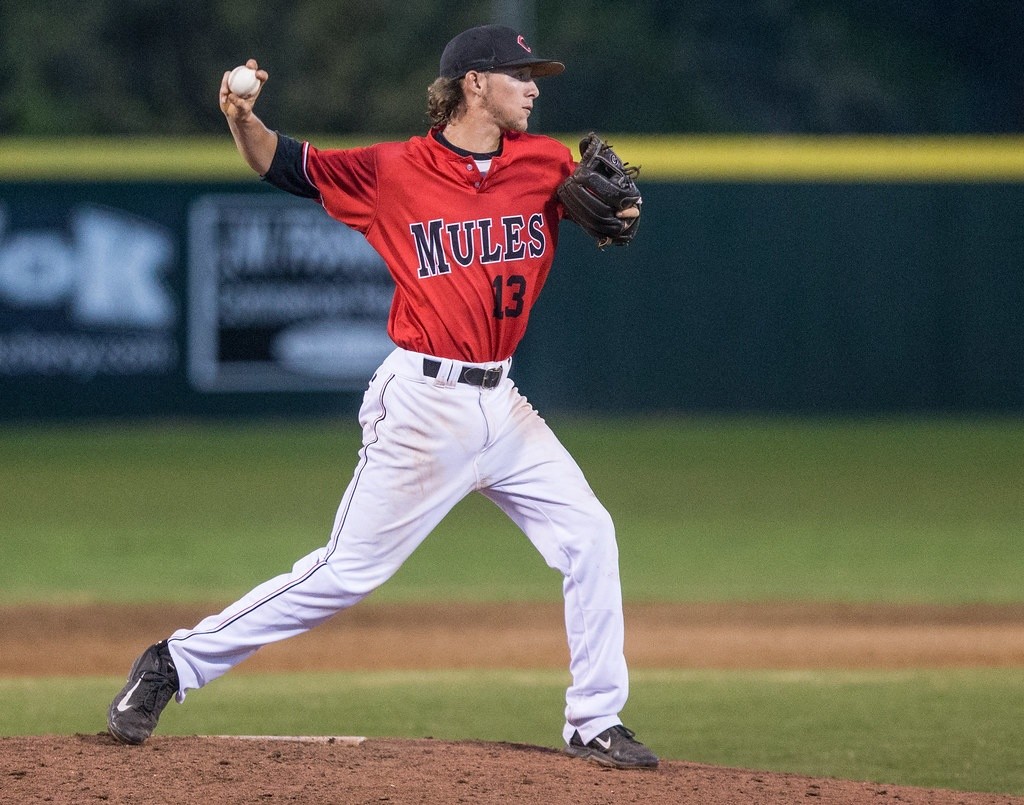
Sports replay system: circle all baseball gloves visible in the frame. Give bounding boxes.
[555,132,641,250]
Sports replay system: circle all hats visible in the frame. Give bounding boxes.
[440,25,565,85]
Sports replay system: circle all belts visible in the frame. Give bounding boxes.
[423,358,510,390]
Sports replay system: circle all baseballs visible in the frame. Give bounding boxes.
[227,65,261,100]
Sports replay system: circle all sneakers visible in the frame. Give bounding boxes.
[570,725,657,769]
[107,639,179,745]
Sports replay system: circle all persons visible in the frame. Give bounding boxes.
[105,22,660,771]
